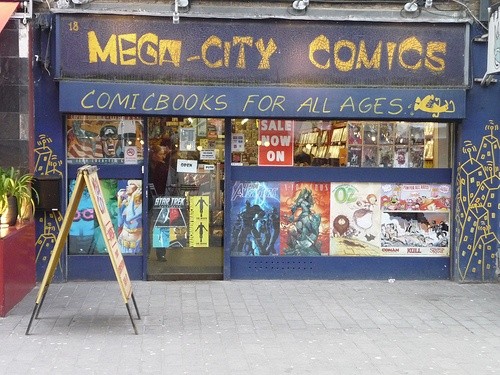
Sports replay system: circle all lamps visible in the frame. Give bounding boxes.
[173,1,181,25]
[296,0,310,8]
[408,0,433,9]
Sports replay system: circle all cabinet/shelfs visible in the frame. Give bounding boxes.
[0,221,36,317]
[294,130,348,166]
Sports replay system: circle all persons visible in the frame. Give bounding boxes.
[148,137,181,262]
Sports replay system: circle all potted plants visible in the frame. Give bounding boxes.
[0,166,40,226]
